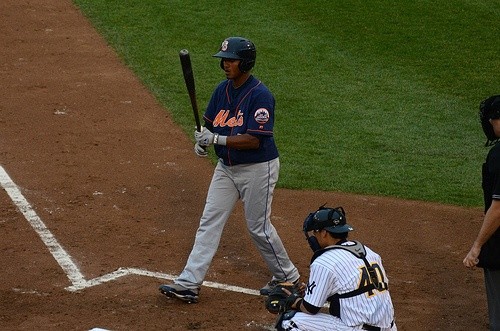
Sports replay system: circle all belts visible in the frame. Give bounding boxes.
[364,320,395,331]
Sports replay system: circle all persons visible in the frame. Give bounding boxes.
[159,37,300,302]
[277,206,398,331]
[463,95,500,331]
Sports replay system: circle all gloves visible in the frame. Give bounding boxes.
[194,125,227,147]
[193,143,210,158]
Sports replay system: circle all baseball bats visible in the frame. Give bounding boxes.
[179,47,206,152]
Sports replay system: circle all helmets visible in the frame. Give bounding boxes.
[302,207,355,235]
[211,37,256,74]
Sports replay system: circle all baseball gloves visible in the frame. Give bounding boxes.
[260,276,307,316]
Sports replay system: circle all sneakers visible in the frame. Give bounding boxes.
[260,274,307,295]
[159,283,200,303]
